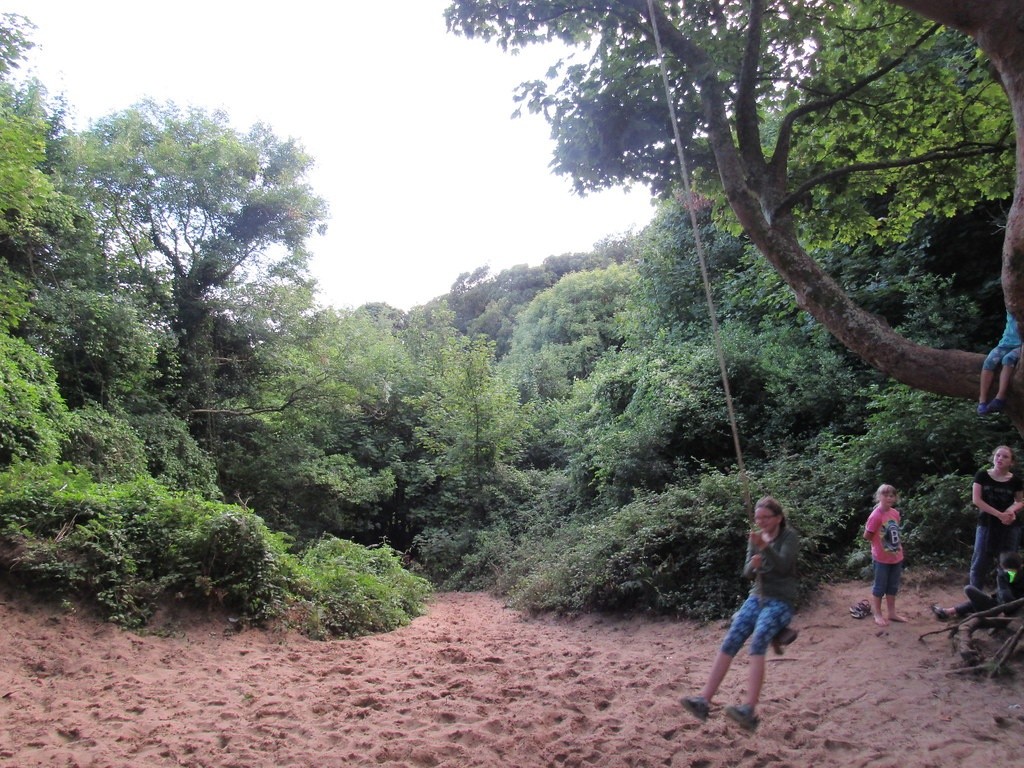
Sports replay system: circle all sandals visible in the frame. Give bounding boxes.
[850,598,871,619]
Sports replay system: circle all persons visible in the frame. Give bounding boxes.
[969,445,1024,592]
[976,310,1021,413]
[863,483,908,626]
[931,551,1024,622]
[678,496,799,731]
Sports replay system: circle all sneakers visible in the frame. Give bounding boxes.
[682,695,710,721]
[725,704,756,731]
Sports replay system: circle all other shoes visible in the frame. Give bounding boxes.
[987,612,1005,636]
[977,398,1008,415]
[930,604,947,619]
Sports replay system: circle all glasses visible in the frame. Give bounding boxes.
[754,514,776,519]
